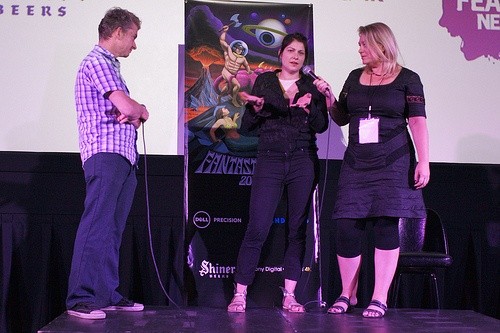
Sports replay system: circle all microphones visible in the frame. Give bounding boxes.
[302,66,330,91]
[140,104,146,123]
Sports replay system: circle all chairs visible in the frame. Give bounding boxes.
[390,207,452,309]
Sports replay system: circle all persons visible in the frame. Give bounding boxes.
[313,21,431,319]
[66,8,149,319]
[227,31,329,316]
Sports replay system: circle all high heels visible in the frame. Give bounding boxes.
[327,289,358,314]
[361,298,388,319]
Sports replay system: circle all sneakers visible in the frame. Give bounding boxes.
[98,296,145,311]
[66,302,106,319]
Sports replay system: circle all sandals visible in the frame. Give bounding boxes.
[279,287,306,313]
[227,289,248,313]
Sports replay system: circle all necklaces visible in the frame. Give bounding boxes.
[370,65,395,76]
